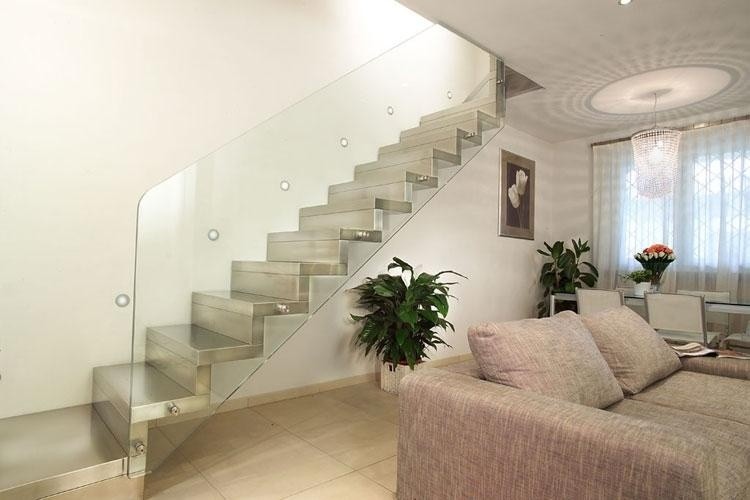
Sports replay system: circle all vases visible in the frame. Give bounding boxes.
[651,272,664,293]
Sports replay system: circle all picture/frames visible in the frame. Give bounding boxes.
[498,147,535,240]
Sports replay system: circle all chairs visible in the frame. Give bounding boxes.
[677,290,731,351]
[724,334,750,353]
[642,291,718,349]
[575,288,626,314]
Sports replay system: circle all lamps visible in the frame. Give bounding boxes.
[628,91,681,197]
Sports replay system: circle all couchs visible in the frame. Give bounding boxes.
[395,337,750,500]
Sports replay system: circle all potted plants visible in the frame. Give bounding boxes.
[346,255,465,392]
[628,270,652,296]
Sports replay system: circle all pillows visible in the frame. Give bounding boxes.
[580,305,684,394]
[468,309,624,408]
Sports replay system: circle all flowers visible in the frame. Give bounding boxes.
[634,243,678,285]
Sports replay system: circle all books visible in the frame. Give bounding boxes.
[669,343,750,359]
